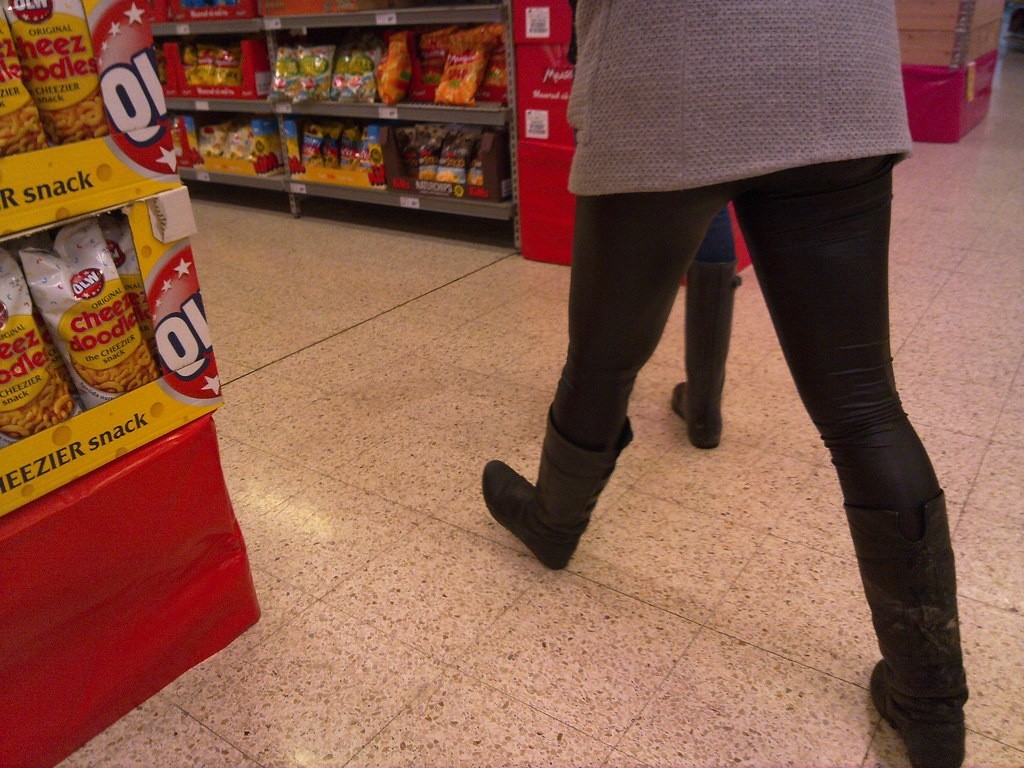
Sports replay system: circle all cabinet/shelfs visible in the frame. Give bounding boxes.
[0,0,225,521]
[146,0,516,221]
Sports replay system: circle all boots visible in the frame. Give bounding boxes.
[481,402,634,570]
[842,490,969,767]
[671,259,742,450]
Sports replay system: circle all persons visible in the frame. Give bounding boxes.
[480,0,972,767]
[671,197,737,453]
